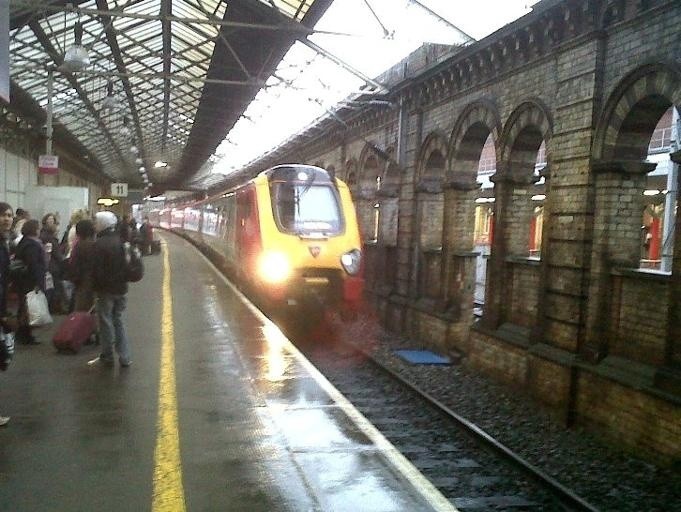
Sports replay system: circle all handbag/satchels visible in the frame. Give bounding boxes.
[124,244,144,283]
[25,289,53,328]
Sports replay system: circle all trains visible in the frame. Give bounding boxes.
[139,164,365,323]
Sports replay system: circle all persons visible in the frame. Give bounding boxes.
[0,200,154,426]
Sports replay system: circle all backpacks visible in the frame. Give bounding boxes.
[43,238,54,273]
[6,241,36,292]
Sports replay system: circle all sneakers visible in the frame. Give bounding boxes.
[1,334,42,367]
[120,360,133,367]
[87,358,115,369]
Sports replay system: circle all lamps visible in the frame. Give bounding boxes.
[62,13,92,64]
[102,77,119,109]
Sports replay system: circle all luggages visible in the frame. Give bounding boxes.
[56,279,76,313]
[52,301,98,355]
